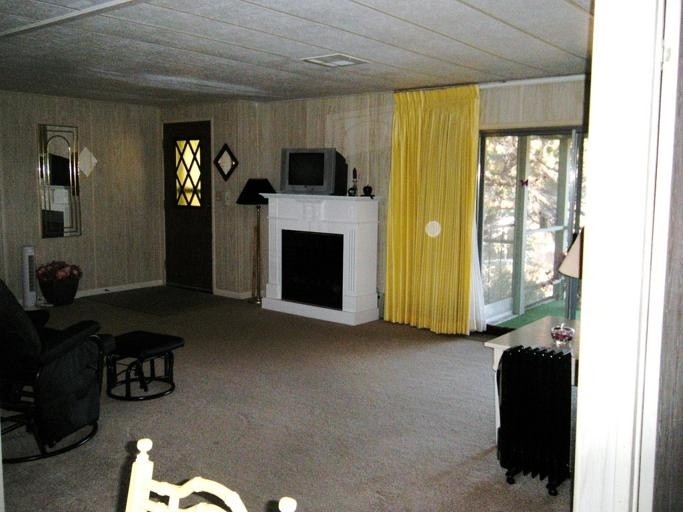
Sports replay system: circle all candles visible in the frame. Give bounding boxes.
[352,167,357,180]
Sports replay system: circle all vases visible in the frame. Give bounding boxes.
[39,280,79,305]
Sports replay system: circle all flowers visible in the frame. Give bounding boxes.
[35,260,81,280]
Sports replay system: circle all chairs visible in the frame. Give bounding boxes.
[0,278,103,463]
[124,438,298,511]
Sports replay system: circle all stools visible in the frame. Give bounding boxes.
[106,330,184,401]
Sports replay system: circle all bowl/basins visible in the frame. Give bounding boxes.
[551,326,574,344]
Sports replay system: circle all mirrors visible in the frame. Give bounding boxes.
[37,124,81,238]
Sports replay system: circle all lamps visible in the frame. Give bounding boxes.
[237,178,277,307]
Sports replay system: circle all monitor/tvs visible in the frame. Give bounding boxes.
[280,147,348,196]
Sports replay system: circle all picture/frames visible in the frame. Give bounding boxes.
[214,143,238,182]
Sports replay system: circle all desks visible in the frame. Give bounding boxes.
[484,314,580,447]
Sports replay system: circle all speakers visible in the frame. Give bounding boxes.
[22,247,40,312]
